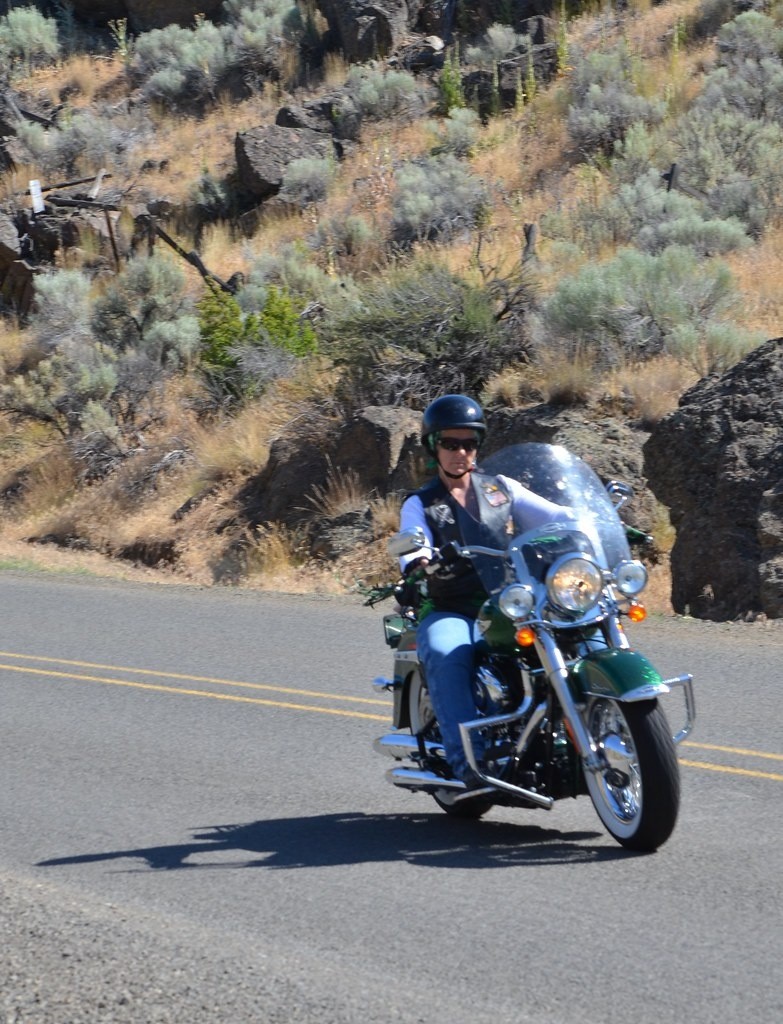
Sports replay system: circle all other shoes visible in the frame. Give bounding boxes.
[462,762,497,791]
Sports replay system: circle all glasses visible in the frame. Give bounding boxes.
[436,438,480,451]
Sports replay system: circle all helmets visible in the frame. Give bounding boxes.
[422,394,485,456]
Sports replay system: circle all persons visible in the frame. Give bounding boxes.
[399,394,624,789]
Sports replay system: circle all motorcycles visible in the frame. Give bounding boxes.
[372,441,696,852]
